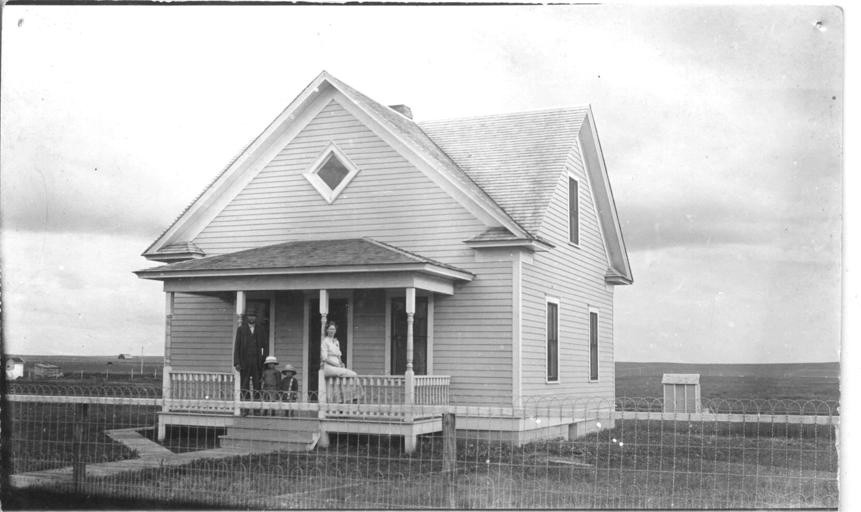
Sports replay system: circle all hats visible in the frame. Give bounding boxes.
[281,365,296,375]
[244,307,257,316]
[263,356,278,364]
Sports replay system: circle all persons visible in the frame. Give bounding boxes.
[234,307,271,416]
[321,320,366,403]
[279,363,300,417]
[258,355,282,417]
